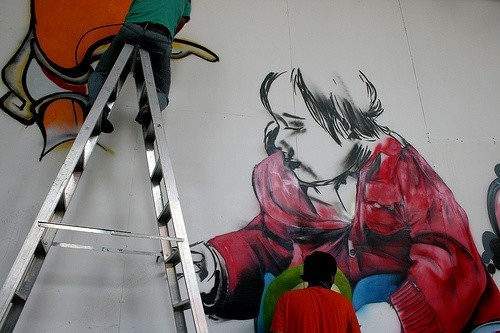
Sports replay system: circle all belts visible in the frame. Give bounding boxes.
[137,21,171,37]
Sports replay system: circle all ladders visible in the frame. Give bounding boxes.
[0,37,209,333]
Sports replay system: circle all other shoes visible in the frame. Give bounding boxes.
[85,106,114,134]
[135,107,152,125]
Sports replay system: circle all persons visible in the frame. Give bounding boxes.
[270,251,361,333]
[85,0,192,133]
